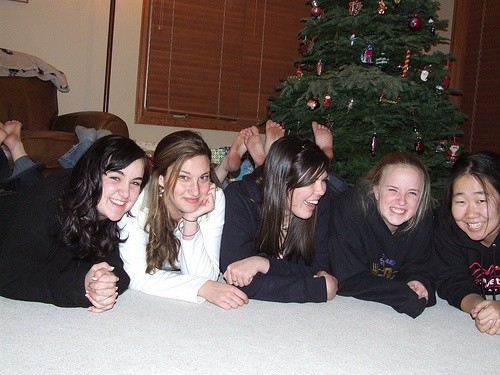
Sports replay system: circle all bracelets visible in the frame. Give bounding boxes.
[182,216,197,222]
[178,221,200,236]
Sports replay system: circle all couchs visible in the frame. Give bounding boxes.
[0,48,129,179]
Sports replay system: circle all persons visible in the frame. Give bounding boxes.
[0,118,150,314]
[264,120,438,318]
[209,125,338,303]
[59,124,249,310]
[432,153,500,335]
